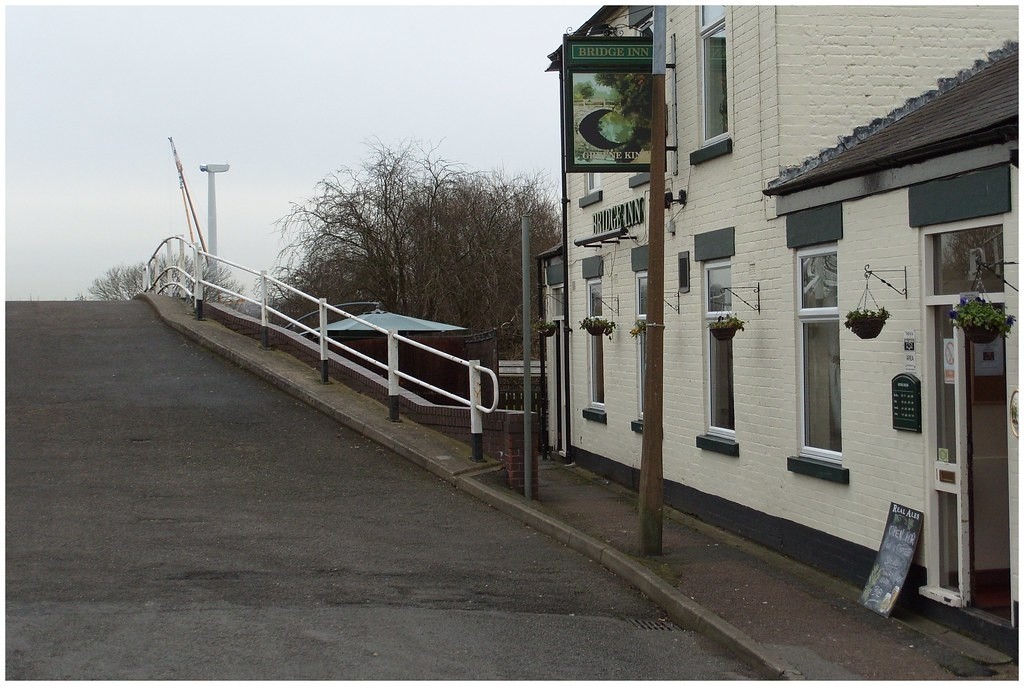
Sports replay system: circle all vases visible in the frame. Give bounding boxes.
[641,327,646,334]
[963,323,999,344]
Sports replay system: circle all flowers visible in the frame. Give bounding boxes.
[628,319,646,339]
[947,295,1017,340]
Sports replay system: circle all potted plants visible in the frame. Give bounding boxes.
[705,313,745,340]
[530,317,556,337]
[843,306,892,340]
[579,315,618,343]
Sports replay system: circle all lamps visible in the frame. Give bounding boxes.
[664,189,686,209]
